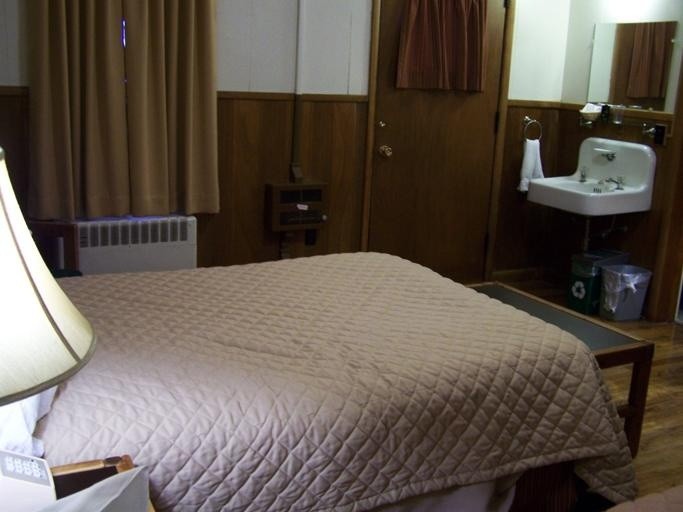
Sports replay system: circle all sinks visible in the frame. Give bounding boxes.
[527,135,658,217]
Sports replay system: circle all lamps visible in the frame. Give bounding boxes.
[0,147,100,404]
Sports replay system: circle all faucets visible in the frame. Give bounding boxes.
[605,174,624,190]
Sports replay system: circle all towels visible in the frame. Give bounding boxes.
[394,0,489,93]
[517,138,545,193]
[625,22,674,99]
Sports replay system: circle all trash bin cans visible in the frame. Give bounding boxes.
[566,247,652,321]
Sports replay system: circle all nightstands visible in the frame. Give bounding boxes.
[49,454,155,510]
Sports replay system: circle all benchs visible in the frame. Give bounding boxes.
[463,280,655,460]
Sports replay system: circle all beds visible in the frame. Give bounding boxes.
[0,249,639,512]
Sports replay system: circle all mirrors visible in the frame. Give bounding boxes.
[586,21,680,115]
